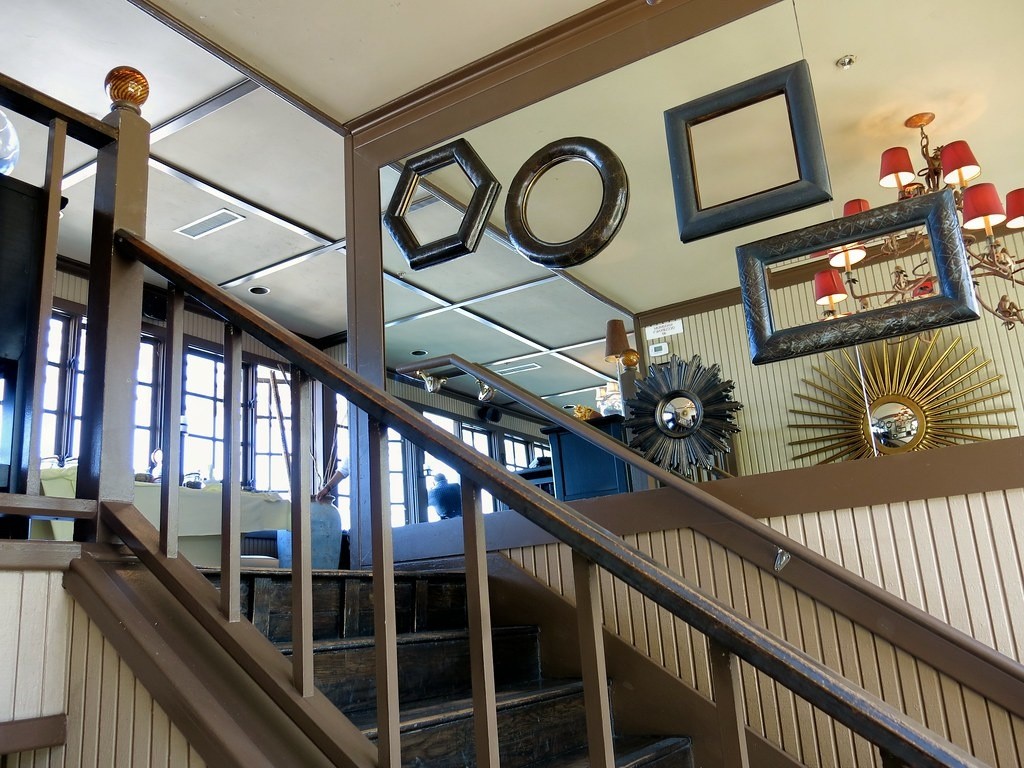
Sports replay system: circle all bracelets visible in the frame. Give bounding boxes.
[324,485,331,493]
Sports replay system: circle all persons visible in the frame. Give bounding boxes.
[316,456,351,503]
[427,473,462,520]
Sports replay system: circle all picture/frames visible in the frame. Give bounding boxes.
[735,186,981,364]
[661,61,833,244]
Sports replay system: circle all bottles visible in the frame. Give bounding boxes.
[64,457,78,468]
[40,455,59,469]
[182,473,202,490]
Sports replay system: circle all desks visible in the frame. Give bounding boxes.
[501,465,553,511]
[28,470,291,567]
[540,415,629,502]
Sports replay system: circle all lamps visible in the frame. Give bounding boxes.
[815,112,1024,345]
[595,382,619,400]
[604,319,630,417]
